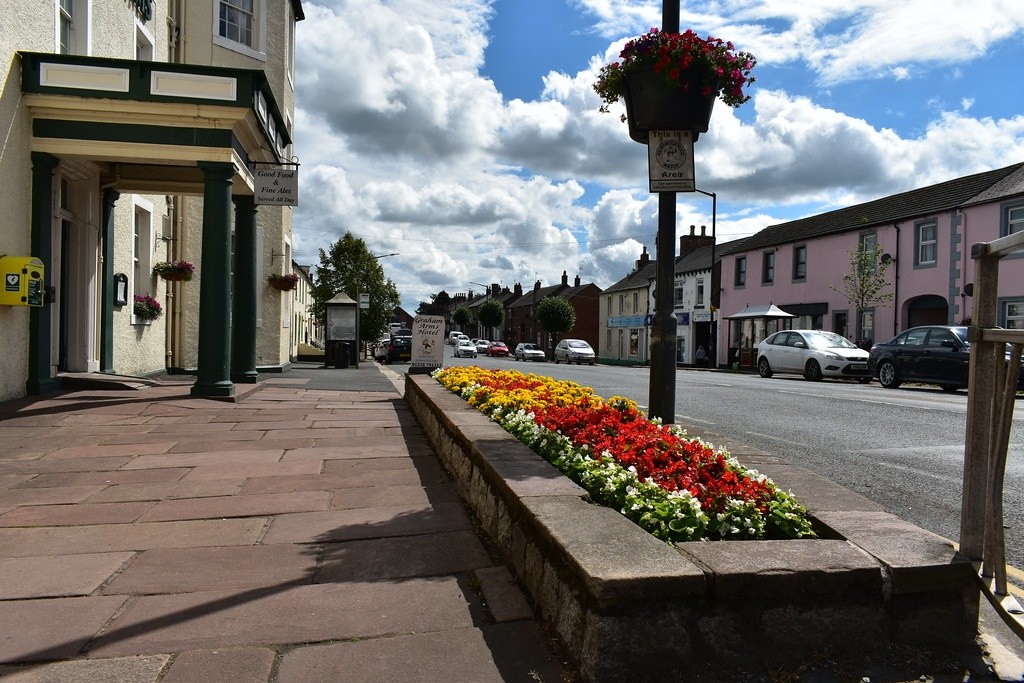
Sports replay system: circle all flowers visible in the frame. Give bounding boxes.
[268,273,298,288]
[593,26,757,124]
[150,261,195,274]
[134,294,162,321]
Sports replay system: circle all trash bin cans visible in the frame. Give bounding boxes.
[335,342,350,369]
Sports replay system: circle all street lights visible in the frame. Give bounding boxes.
[352,267,365,370]
[467,282,489,340]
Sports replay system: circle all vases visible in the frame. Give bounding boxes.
[160,273,185,281]
[272,280,288,290]
[624,90,716,144]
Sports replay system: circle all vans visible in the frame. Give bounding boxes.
[376,334,390,341]
[398,329,411,336]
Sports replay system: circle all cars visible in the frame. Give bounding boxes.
[370,339,401,363]
[449,331,510,357]
[454,341,477,358]
[555,339,594,365]
[866,325,1024,392]
[756,330,870,384]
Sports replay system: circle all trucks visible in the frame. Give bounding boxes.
[387,323,401,334]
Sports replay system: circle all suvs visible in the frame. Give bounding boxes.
[385,335,413,364]
[514,343,545,362]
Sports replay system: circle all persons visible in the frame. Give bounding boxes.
[696,346,706,359]
